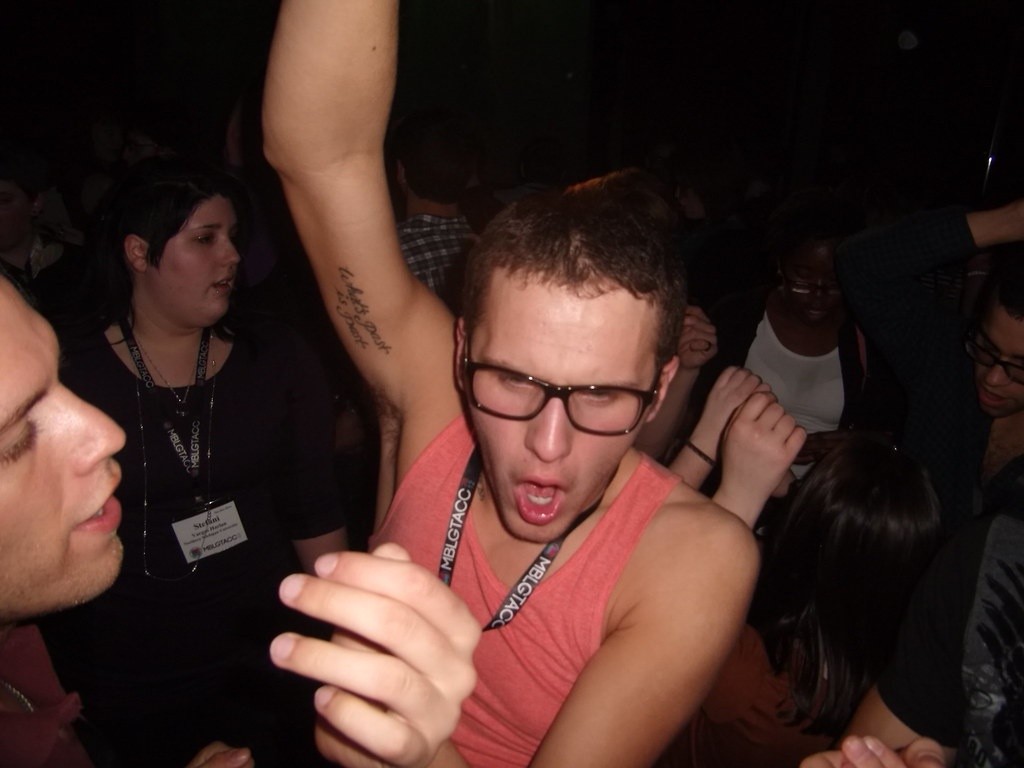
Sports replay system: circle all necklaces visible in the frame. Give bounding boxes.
[138,339,196,416]
[139,338,215,582]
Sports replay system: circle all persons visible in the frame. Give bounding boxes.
[0,0,1024,767]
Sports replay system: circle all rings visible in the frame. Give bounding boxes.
[58,231,64,235]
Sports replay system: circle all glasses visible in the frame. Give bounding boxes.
[966,326,1024,382]
[776,259,844,297]
[461,327,667,437]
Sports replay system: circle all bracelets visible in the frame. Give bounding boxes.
[686,440,716,465]
